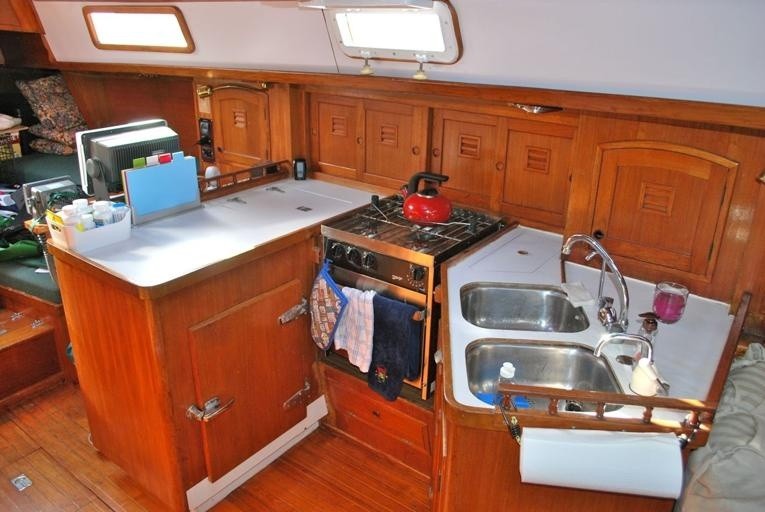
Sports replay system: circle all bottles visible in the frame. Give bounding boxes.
[60,199,128,232]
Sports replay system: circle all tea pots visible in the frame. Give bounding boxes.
[403,172,452,227]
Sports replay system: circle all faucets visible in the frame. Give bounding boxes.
[562,232,629,334]
[593,332,654,366]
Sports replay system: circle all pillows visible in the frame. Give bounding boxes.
[15,80,53,128]
[26,140,78,156]
[28,75,86,131]
[27,123,90,145]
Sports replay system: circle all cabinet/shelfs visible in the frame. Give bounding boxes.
[426,312,455,512]
[49,231,318,511]
[563,114,765,303]
[428,107,578,234]
[193,75,270,165]
[315,362,436,485]
[306,92,424,197]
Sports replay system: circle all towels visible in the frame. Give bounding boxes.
[333,288,377,374]
[310,269,348,350]
[369,295,421,402]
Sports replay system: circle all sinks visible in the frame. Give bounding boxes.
[464,337,625,412]
[459,281,590,333]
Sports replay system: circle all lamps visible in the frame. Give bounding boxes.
[411,54,426,80]
[358,49,370,75]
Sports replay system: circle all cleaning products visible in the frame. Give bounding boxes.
[493,362,520,407]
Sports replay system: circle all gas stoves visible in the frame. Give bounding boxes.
[320,193,508,295]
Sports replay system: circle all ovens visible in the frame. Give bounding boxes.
[318,262,433,401]
